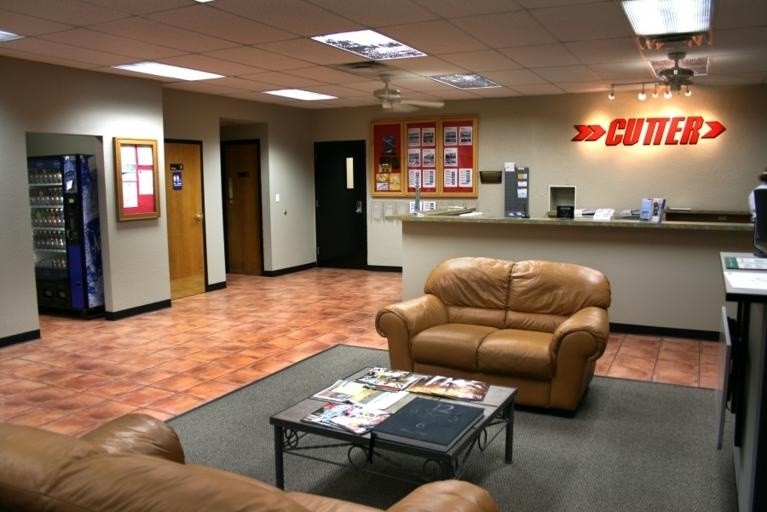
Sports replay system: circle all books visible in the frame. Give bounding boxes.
[299,366,490,453]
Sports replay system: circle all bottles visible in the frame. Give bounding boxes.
[31,208,65,249]
[48,186,63,205]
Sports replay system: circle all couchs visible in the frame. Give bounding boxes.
[378,258,610,411]
[3,415,498,512]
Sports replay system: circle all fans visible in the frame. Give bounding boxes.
[372,70,445,114]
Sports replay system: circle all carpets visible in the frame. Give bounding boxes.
[165,343,739,511]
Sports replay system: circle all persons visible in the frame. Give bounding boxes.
[747,172,767,221]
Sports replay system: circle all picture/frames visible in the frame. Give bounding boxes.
[371,115,479,198]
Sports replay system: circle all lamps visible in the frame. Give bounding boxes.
[619,0,712,92]
